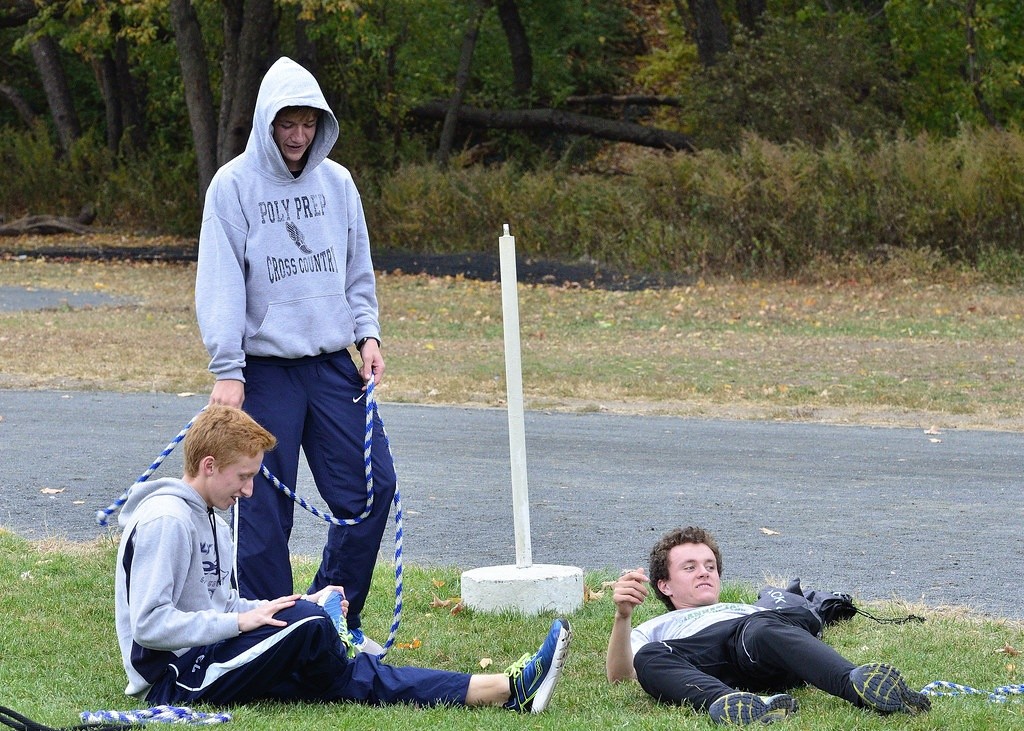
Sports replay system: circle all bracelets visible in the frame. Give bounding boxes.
[358,337,381,351]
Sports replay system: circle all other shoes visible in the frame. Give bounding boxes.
[710,693,799,728]
[849,663,932,715]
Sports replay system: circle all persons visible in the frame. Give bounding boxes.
[606,524,931,725]
[115,404,569,714]
[193,56,396,656]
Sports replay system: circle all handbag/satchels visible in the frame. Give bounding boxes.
[755,576,924,637]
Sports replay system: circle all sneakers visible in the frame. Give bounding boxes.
[346,628,387,660]
[317,589,353,659]
[503,618,568,714]
